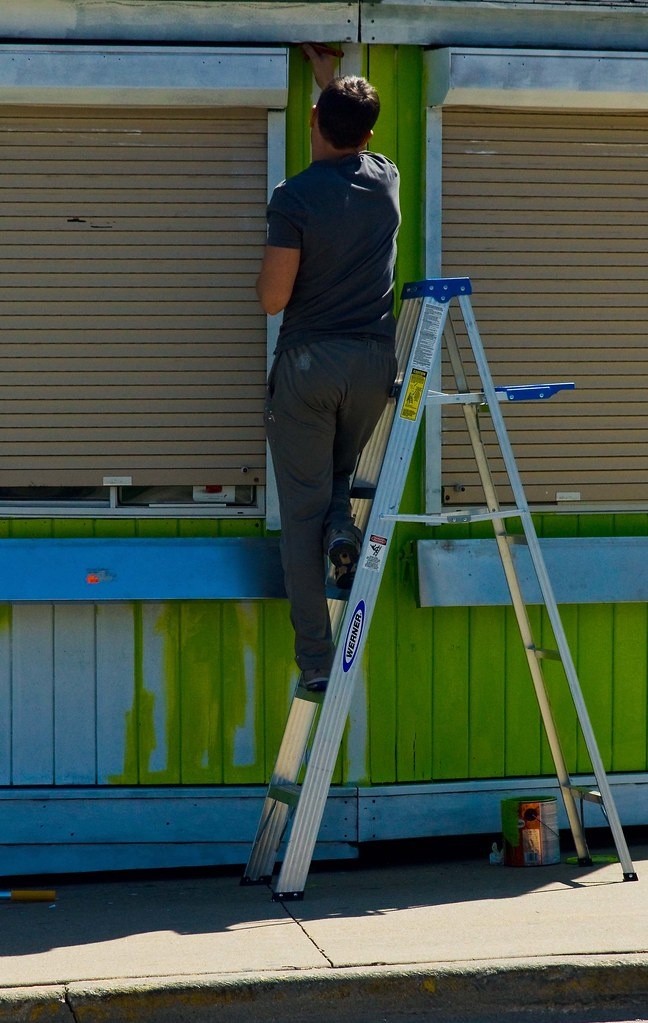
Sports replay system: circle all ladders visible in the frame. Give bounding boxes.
[240,276,638,903]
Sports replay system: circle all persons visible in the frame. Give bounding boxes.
[254,43,402,696]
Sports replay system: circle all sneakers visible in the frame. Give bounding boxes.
[326,528,362,590]
[297,663,334,691]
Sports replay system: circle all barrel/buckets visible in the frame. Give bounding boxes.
[500,796,561,866]
